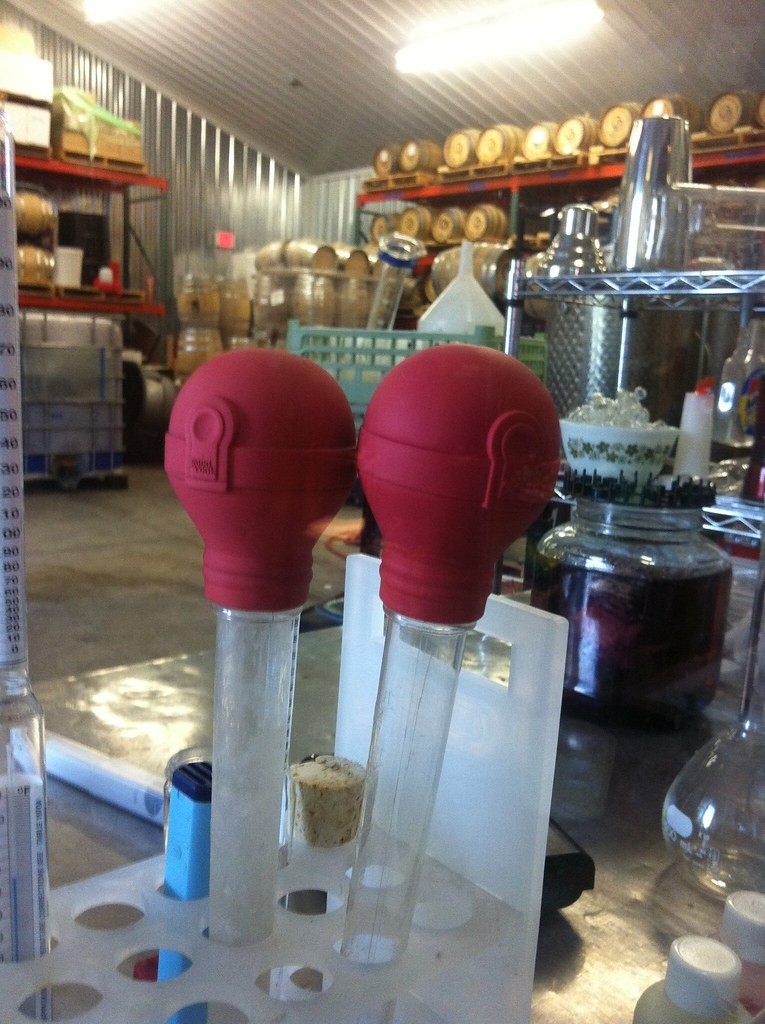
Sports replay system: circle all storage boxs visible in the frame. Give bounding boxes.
[0,49,148,175]
[287,319,548,438]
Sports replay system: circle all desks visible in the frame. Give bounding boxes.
[0,555,765,1024]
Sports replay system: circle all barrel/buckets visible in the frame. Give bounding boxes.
[18,246,55,280]
[171,87,765,381]
[16,191,59,238]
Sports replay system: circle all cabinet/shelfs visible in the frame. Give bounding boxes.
[342,131,765,720]
[0,153,167,490]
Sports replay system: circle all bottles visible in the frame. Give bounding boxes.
[674,387,714,480]
[631,935,753,1024]
[530,498,733,721]
[667,518,765,897]
[710,320,765,447]
[704,890,765,1017]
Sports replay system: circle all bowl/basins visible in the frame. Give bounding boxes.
[558,419,679,487]
